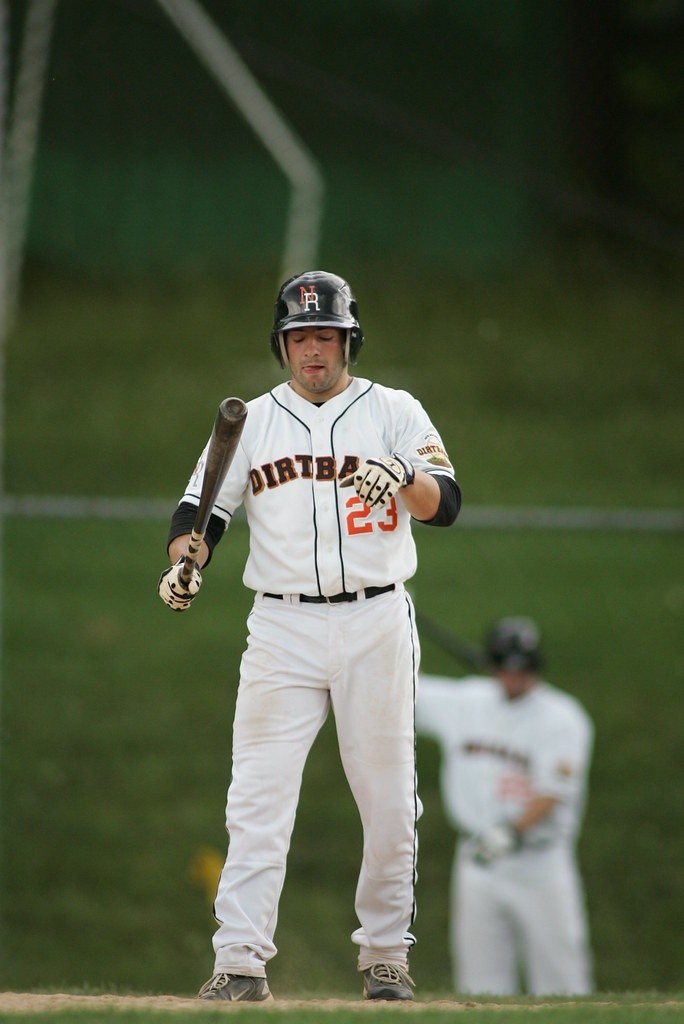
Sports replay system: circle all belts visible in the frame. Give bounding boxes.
[263,583,395,605]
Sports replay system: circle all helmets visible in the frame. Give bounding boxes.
[270,270,364,366]
[487,616,540,670]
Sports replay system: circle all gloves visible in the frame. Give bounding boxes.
[472,825,522,867]
[158,554,202,612]
[338,452,415,510]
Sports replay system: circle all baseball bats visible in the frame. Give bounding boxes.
[180,395,246,584]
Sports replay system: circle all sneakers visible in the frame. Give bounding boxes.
[196,973,275,1002]
[362,963,416,1000]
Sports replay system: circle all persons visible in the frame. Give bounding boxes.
[160,270,462,1003]
[415,612,598,1000]
